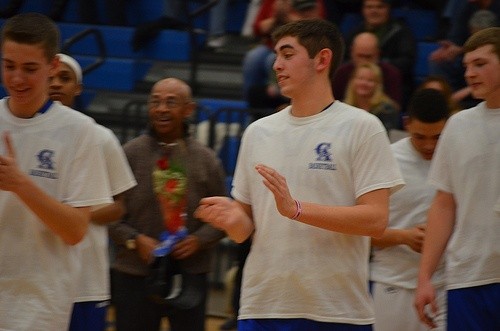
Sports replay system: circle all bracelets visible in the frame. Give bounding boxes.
[291,199,301,220]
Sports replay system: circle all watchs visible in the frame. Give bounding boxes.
[126,235,144,255]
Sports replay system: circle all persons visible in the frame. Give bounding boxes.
[194,0,500,331]
[47,52,139,331]
[107,78,229,331]
[0,14,115,331]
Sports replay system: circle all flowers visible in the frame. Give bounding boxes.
[152,152,189,299]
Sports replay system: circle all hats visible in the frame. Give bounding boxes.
[57,52,83,83]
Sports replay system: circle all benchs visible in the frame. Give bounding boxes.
[340,9,441,80]
[0,0,252,195]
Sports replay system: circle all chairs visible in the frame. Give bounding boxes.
[122,100,212,148]
[212,107,270,177]
